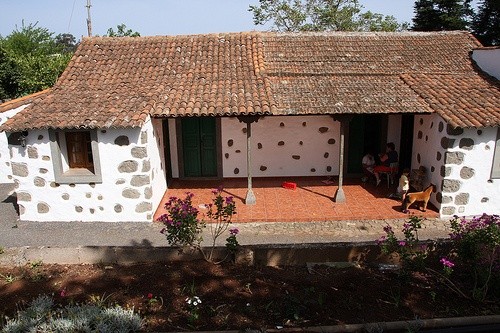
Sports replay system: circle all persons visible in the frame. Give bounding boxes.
[373,142,399,187]
[361,144,381,187]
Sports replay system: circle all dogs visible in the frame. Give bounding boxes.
[400,184,436,212]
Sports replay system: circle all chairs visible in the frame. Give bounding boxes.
[379,163,398,188]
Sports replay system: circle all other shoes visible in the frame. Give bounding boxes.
[376,178,381,187]
[390,180,393,184]
